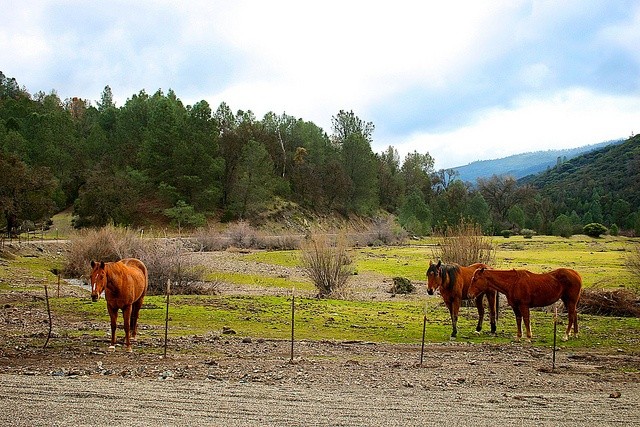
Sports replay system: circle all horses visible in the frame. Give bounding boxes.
[425,260,500,338]
[88,257,149,353]
[466,265,583,343]
